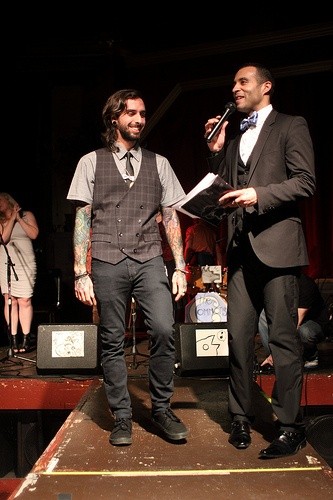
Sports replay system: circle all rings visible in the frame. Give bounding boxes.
[183,291,186,293]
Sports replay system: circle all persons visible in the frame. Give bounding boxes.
[0,192,39,354]
[185,205,220,265]
[67,89,189,444]
[257,275,330,367]
[204,63,315,458]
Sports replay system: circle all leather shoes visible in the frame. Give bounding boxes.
[258,430,307,457]
[228,421,252,448]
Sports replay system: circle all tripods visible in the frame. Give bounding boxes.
[122,296,149,368]
[0,235,36,363]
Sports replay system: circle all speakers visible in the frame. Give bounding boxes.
[36,322,99,375]
[174,323,231,377]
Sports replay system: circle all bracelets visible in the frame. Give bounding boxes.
[75,274,87,280]
[176,268,186,275]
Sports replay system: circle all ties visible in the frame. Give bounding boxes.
[125,152,134,187]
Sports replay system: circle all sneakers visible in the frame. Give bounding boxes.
[153,409,188,440]
[109,418,132,445]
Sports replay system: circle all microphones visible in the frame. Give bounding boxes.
[205,102,236,142]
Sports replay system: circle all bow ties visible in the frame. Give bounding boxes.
[239,111,258,131]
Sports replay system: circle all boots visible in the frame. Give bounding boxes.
[11,333,19,353]
[19,333,30,353]
